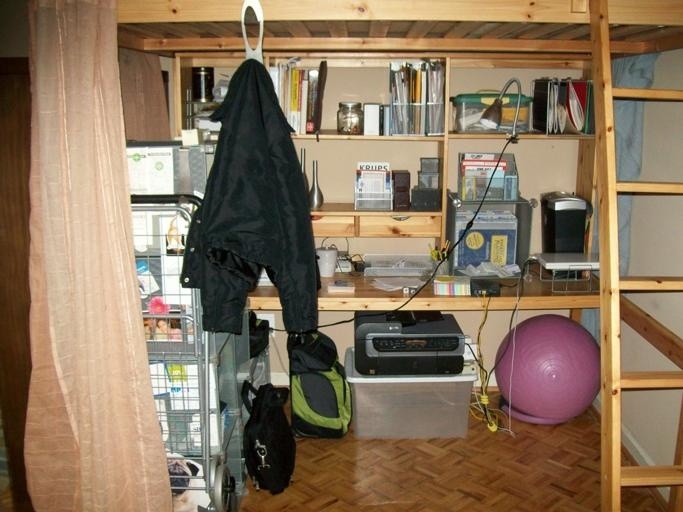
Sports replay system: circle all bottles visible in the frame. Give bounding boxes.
[336,101,364,135]
[192,66,214,102]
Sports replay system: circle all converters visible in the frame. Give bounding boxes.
[355,261,371,272]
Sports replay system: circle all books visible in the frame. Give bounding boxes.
[458,152,518,202]
[386,59,445,138]
[325,279,356,294]
[353,161,392,209]
[122,138,228,512]
[275,62,319,137]
[534,76,587,135]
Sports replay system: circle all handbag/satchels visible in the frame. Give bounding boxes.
[241,380,296,494]
[287,331,352,438]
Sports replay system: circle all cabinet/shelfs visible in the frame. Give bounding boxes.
[169,53,601,311]
[132,206,238,512]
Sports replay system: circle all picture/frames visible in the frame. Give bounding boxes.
[125,140,182,194]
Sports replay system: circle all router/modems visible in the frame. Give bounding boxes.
[471,279,500,296]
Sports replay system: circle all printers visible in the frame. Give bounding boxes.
[354,310,465,374]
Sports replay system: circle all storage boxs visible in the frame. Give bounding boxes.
[344,346,477,438]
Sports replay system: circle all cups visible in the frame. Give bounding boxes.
[316,247,337,278]
